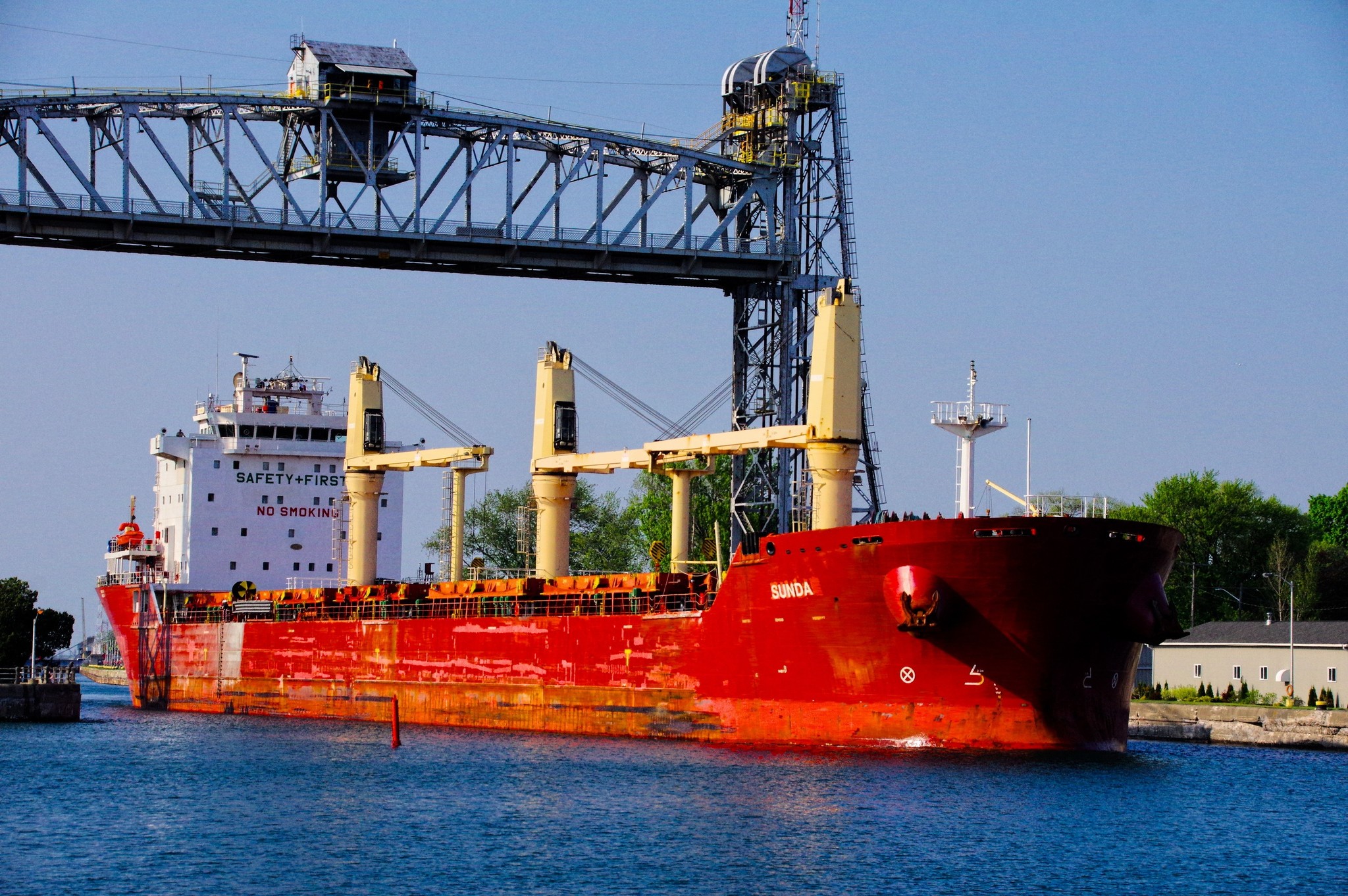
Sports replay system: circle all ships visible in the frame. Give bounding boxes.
[98,276,1181,761]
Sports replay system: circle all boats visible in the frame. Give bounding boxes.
[79,638,128,687]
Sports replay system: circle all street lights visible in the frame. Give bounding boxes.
[1214,582,1242,620]
[1263,572,1294,700]
[31,606,43,678]
